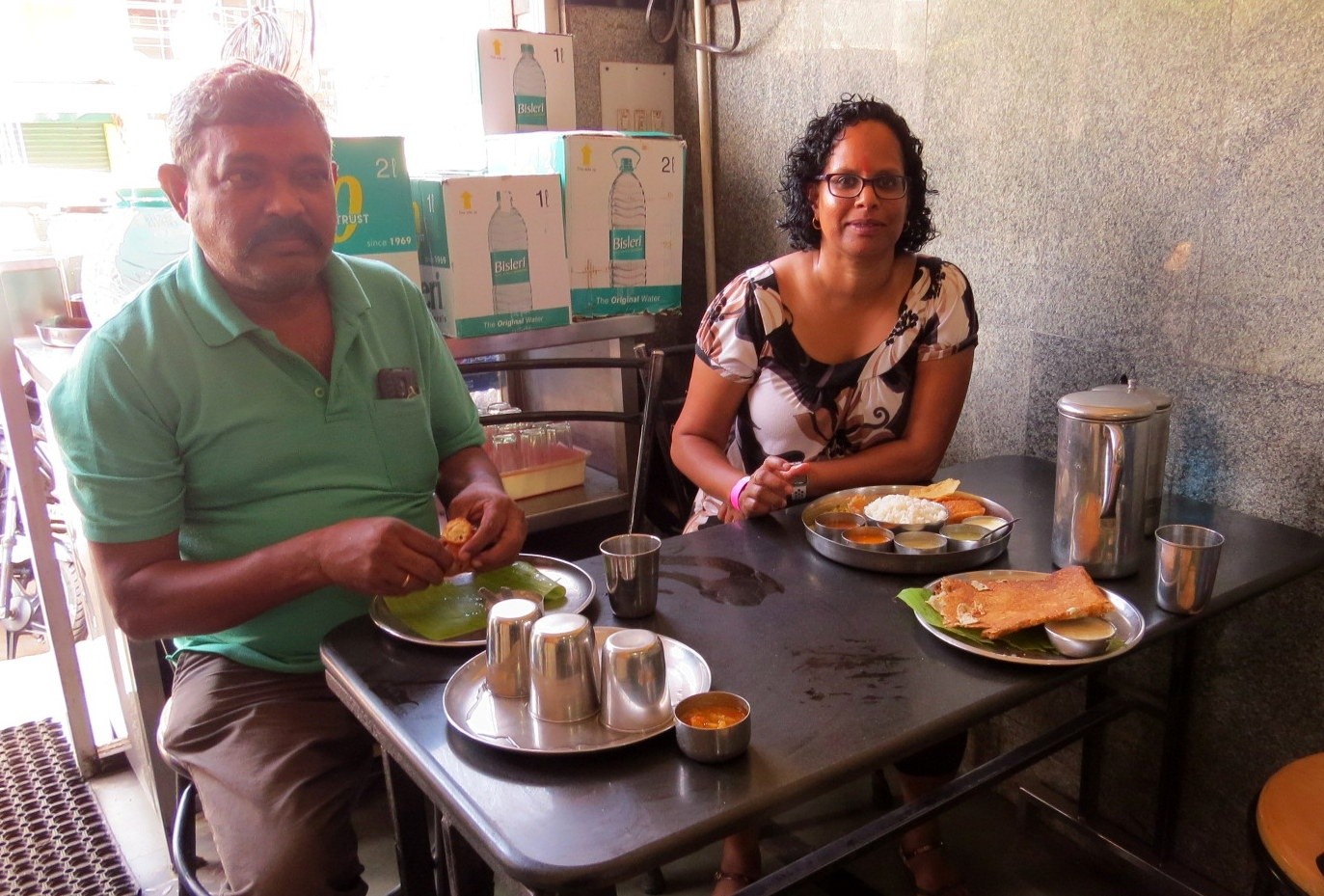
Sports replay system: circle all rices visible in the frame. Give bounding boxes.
[863,494,947,525]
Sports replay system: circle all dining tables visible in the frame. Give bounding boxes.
[319,455,1324,895]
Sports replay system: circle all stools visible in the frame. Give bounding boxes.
[1247,751,1324,896]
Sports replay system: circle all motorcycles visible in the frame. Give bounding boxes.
[0,379,91,662]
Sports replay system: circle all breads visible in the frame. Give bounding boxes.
[444,518,477,561]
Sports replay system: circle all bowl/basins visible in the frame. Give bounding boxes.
[1044,617,1117,658]
[674,692,751,763]
[894,516,1010,554]
[815,512,895,553]
[864,501,948,534]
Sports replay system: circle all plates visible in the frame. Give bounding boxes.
[368,554,597,647]
[443,626,711,753]
[914,570,1146,666]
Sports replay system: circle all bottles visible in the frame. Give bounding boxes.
[609,146,647,287]
[513,44,548,132]
[488,191,532,313]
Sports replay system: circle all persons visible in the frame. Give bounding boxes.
[49,65,527,896]
[671,92,979,535]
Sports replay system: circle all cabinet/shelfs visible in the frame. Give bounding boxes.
[15,315,658,868]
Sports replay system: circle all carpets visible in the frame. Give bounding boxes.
[0,716,143,896]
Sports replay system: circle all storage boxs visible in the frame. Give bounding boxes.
[118,137,420,291]
[409,171,574,339]
[488,130,683,317]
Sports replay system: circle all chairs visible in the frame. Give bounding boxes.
[634,338,897,810]
[155,637,453,896]
[458,347,663,532]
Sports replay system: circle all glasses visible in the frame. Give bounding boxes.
[812,172,911,200]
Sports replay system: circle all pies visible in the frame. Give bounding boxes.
[926,565,1113,640]
[908,478,985,524]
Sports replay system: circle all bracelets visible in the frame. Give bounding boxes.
[789,461,807,500]
[730,476,750,511]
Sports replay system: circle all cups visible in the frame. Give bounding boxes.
[478,402,572,472]
[486,599,673,733]
[1155,524,1225,615]
[599,534,662,619]
[1051,379,1175,579]
